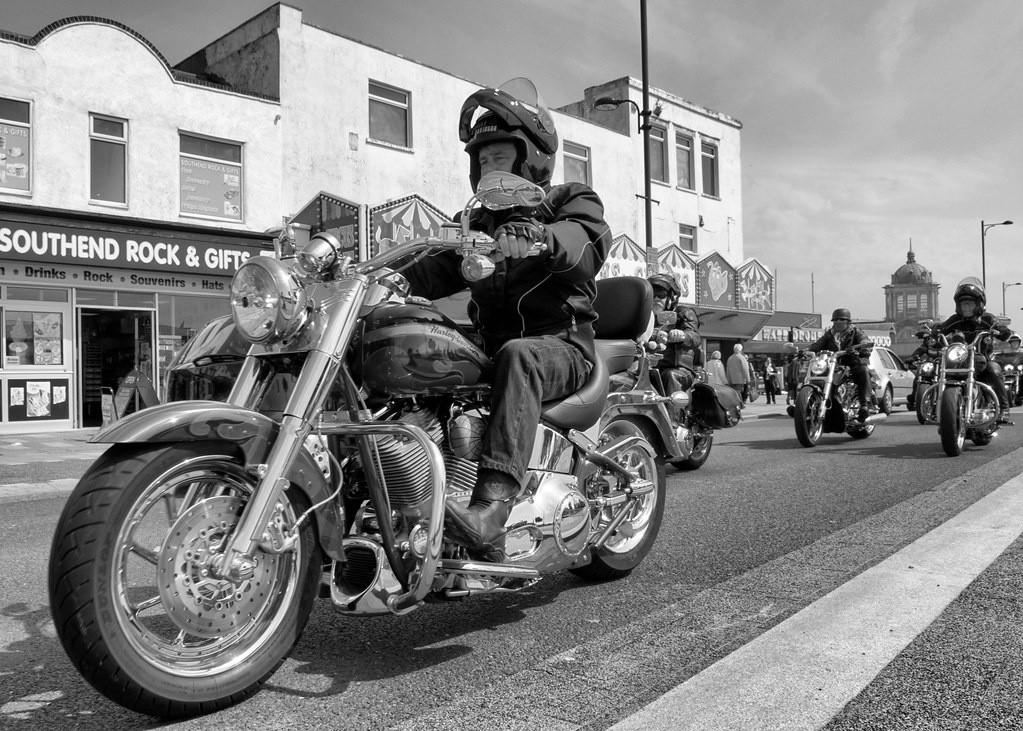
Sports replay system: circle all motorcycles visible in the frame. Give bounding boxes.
[785,342,878,449]
[51,221,675,724]
[603,272,716,471]
[994,352,1023,408]
[911,313,1014,457]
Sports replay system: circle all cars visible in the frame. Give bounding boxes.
[870,347,915,415]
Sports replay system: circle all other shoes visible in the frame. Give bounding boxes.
[858,401,870,419]
[999,408,1010,421]
[787,406,796,417]
[766,401,776,404]
[907,391,915,402]
[787,397,796,404]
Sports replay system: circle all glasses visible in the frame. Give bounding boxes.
[834,319,847,321]
[654,288,668,299]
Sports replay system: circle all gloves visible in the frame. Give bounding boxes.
[995,327,1008,338]
[916,329,930,339]
[797,348,808,358]
[905,356,915,364]
[859,348,870,359]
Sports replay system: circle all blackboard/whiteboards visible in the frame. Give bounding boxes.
[107,370,161,424]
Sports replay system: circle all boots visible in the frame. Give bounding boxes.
[444,471,520,562]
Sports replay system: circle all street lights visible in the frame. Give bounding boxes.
[980,218,1014,287]
[1003,282,1022,317]
[595,93,655,277]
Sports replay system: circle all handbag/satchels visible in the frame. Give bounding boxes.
[693,383,746,428]
[749,386,759,403]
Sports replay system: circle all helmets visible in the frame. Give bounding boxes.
[831,308,851,322]
[459,88,558,202]
[954,284,986,315]
[647,274,681,311]
[1008,334,1022,347]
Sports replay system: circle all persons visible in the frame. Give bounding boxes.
[391,78,613,561]
[645,274,703,428]
[797,308,873,418]
[742,354,755,404]
[704,350,728,386]
[903,275,1023,424]
[761,357,778,404]
[726,344,751,408]
[785,354,801,405]
